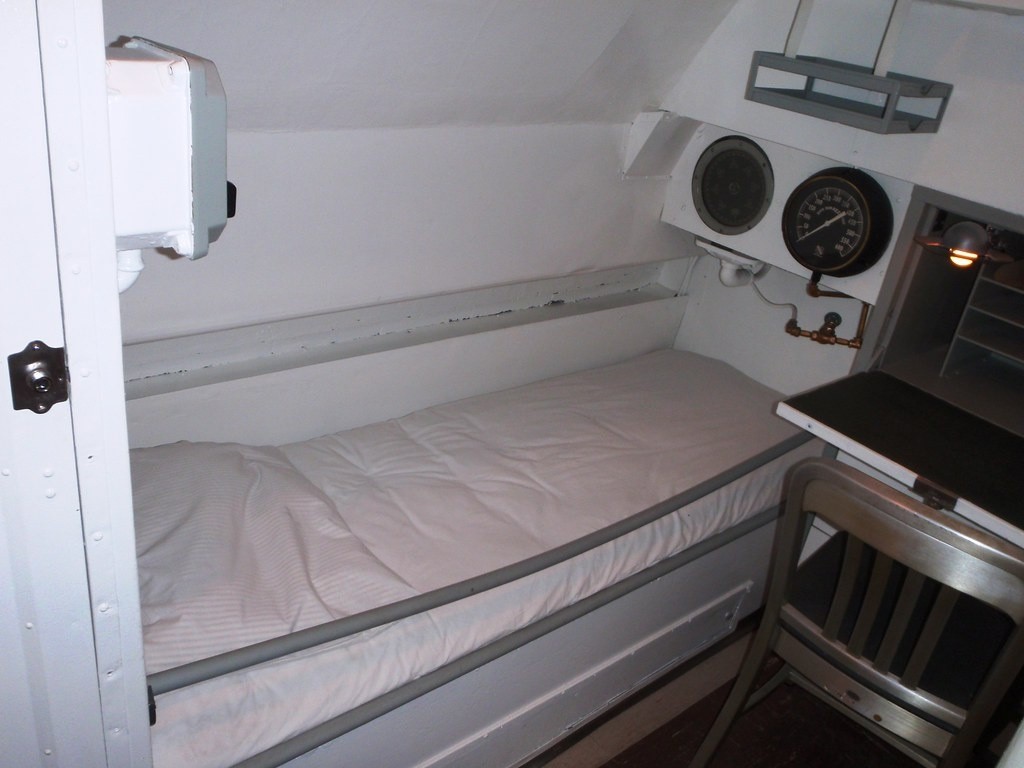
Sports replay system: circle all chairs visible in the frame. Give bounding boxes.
[689,457,1024,768]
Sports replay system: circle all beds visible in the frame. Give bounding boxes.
[130,347,826,768]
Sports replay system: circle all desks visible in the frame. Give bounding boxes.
[774,364,1024,550]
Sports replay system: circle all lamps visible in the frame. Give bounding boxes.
[913,221,1016,270]
